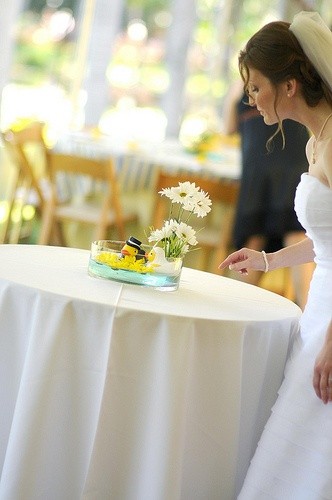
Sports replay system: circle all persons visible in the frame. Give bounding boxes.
[218,8,332,500]
[226,87,310,258]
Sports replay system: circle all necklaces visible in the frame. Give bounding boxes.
[311,114,331,164]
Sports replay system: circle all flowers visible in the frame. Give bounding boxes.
[95,252,158,275]
[147,181,213,262]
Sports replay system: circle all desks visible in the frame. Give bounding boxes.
[0,243,302,500]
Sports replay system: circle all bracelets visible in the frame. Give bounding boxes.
[263,249,270,274]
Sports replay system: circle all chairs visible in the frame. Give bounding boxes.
[38,146,138,249]
[151,171,241,277]
[0,120,66,247]
